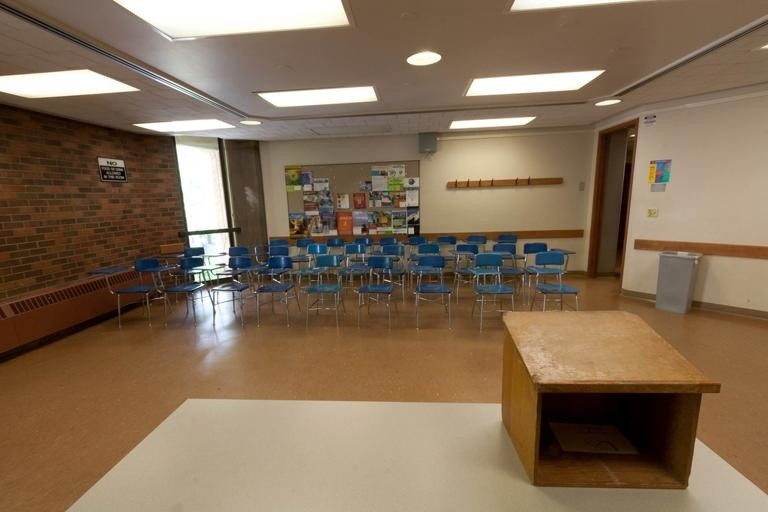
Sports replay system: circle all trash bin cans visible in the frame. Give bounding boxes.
[655,250,704,314]
